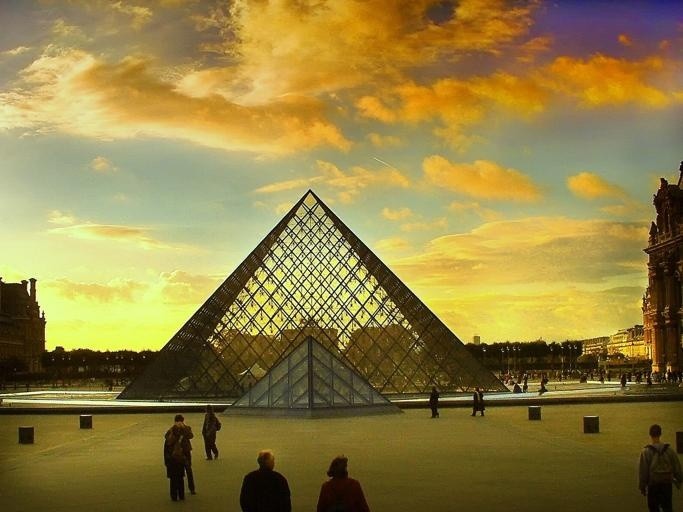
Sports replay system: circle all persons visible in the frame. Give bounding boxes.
[639,425,682,511]
[201,405,220,459]
[240,449,291,511]
[163,425,183,500]
[498,368,683,392]
[470,386,485,416]
[428,386,439,418]
[316,454,369,511]
[164,414,196,495]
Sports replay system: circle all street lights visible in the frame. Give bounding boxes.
[12,366,16,391]
[560,346,565,377]
[617,355,621,379]
[481,347,486,368]
[550,346,554,370]
[82,357,86,386]
[104,354,145,384]
[68,356,72,386]
[50,357,55,387]
[500,344,522,371]
[61,356,65,387]
[567,343,572,373]
[624,354,631,372]
[573,343,578,372]
[606,354,611,382]
[636,353,641,371]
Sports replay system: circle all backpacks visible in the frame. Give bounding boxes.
[645,443,672,484]
[210,416,221,431]
